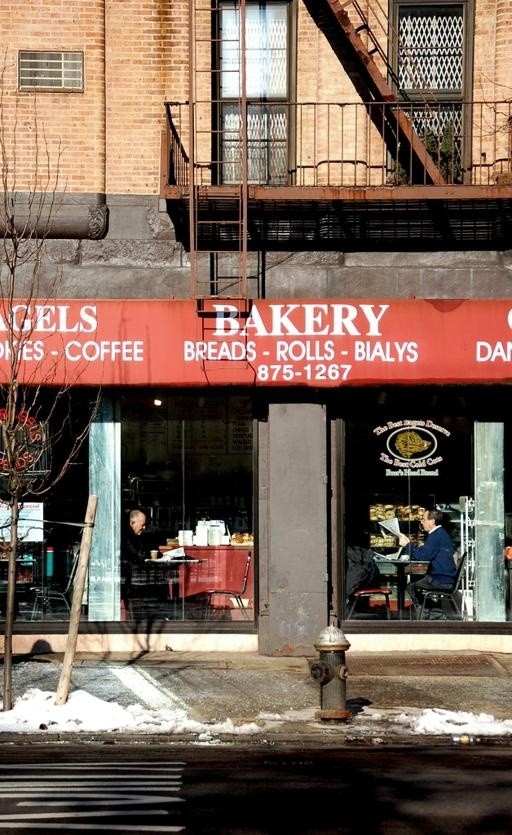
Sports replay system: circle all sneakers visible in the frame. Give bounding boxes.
[415,605,432,620]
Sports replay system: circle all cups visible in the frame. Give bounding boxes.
[150,550,158,560]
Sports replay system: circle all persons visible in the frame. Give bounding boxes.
[396,508,456,621]
[121,510,168,615]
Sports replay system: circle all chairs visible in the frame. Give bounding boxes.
[345,546,392,619]
[204,551,250,620]
[31,551,80,620]
[418,553,467,620]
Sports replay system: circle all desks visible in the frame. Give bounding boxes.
[145,559,208,619]
[375,560,431,619]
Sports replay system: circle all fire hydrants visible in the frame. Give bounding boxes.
[311,621,352,724]
